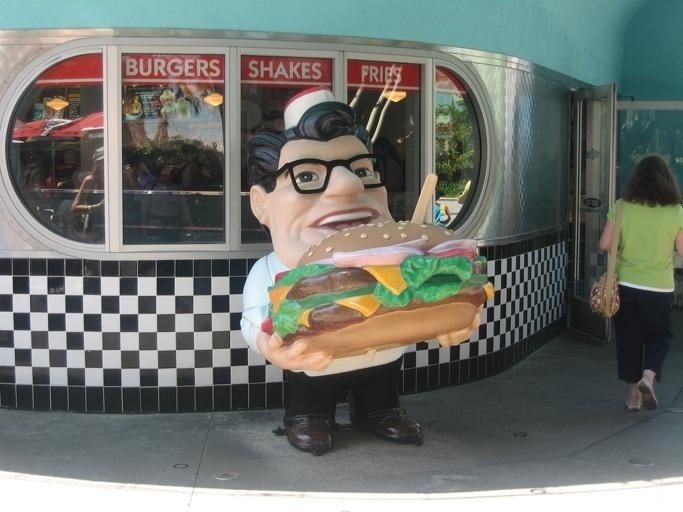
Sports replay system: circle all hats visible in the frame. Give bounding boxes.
[93,148,104,162]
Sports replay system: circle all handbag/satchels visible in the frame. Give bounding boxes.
[590,272,621,317]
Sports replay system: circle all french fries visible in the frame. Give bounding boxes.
[127,103,142,114]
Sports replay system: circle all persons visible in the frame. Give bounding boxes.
[596,153,683,416]
[373,138,417,220]
[135,165,191,241]
[238,83,428,456]
[436,111,462,194]
[66,147,105,241]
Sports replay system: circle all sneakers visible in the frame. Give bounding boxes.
[625,400,640,410]
[638,380,658,409]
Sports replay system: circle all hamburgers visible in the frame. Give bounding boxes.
[259,221,495,360]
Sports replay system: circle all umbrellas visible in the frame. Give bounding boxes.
[10,118,82,186]
[65,109,103,141]
